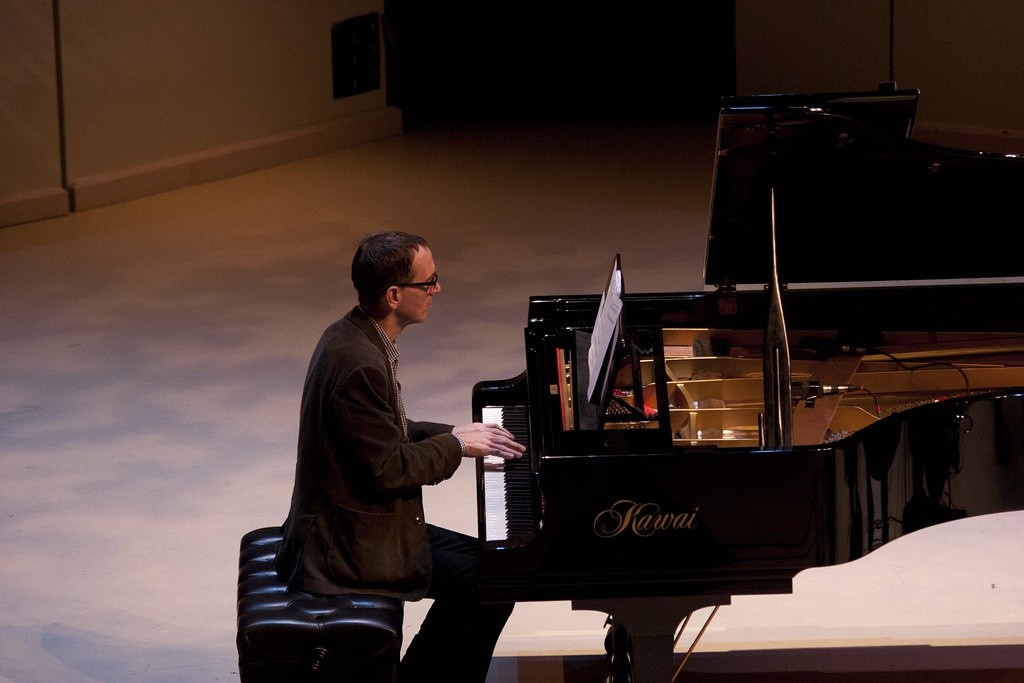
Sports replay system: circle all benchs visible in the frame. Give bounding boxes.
[236,526,407,683]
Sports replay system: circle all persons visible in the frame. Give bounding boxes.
[278,231,527,683]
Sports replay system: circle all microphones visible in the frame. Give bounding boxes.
[798,335,869,357]
[784,380,860,400]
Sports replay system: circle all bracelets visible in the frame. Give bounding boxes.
[451,432,467,457]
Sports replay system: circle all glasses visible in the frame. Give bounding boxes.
[395,273,438,287]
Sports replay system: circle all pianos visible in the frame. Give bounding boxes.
[471,87,1024,683]
